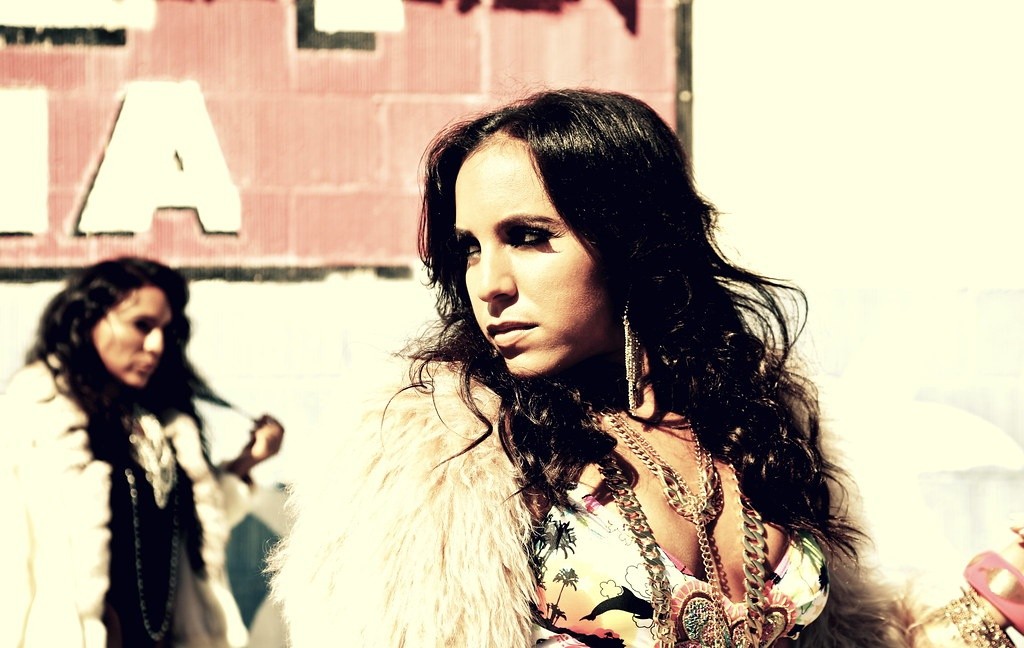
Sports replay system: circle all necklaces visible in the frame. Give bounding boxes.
[124,469,185,642]
[591,396,766,648]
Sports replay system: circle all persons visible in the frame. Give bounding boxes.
[0,255,286,648]
[257,87,1024,648]
